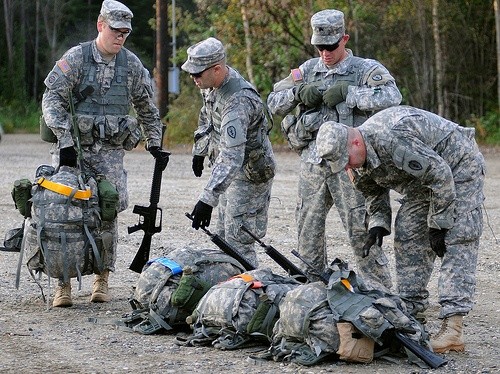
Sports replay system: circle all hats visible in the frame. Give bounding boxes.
[311,10,346,45]
[316,121,349,172]
[100,0,133,30]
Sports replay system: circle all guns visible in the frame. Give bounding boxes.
[239,223,311,285]
[185,212,258,271]
[127,122,172,275]
[290,249,450,369]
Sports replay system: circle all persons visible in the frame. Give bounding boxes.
[42,0,172,306]
[317,105,486,354]
[181,37,276,268]
[268,9,402,286]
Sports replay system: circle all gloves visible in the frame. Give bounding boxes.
[323,84,348,108]
[191,200,213,230]
[299,85,324,108]
[428,228,448,256]
[360,226,386,259]
[150,145,169,170]
[60,146,78,167]
[192,156,205,177]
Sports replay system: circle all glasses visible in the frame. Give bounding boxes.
[191,64,219,78]
[316,35,344,52]
[181,37,226,73]
[109,25,130,39]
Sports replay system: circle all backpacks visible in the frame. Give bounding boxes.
[134,247,431,365]
[23,164,114,278]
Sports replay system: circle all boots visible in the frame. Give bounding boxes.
[52,279,74,308]
[91,270,110,303]
[430,315,465,353]
